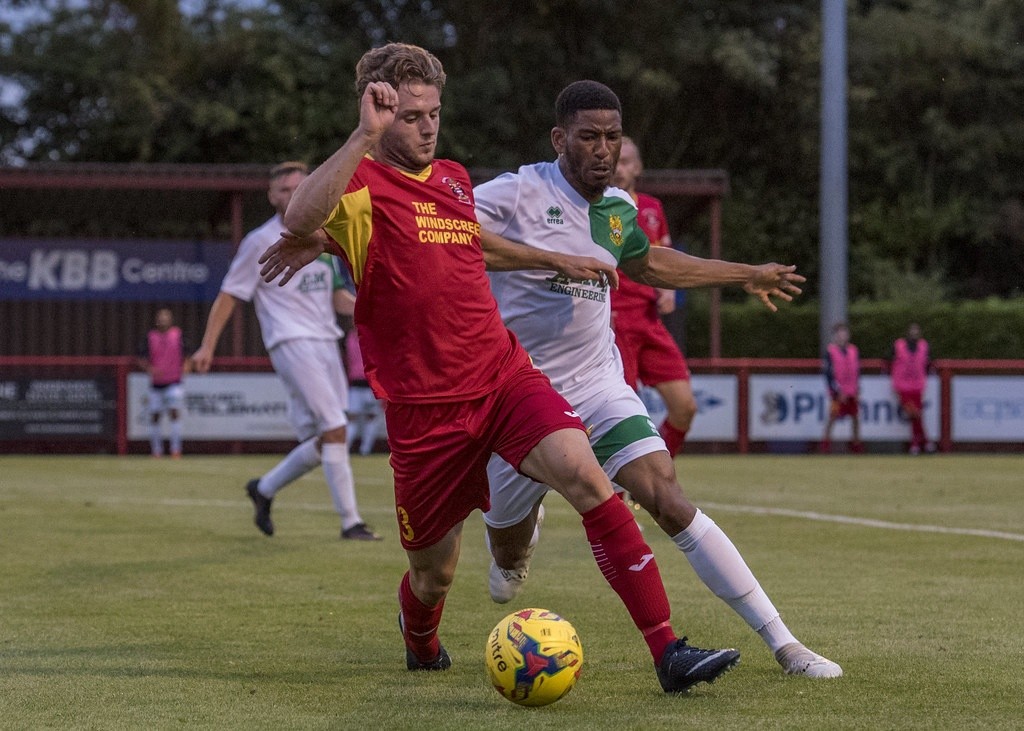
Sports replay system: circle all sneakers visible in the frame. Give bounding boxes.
[653,634,741,693]
[245,478,275,535]
[489,548,534,604]
[398,608,452,670]
[776,643,843,678]
[340,518,384,540]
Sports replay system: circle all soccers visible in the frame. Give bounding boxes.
[485,606,584,708]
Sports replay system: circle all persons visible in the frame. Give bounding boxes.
[611,137,697,459]
[283,43,741,695]
[885,320,935,456]
[339,318,379,453]
[190,161,382,542]
[820,325,863,455]
[140,308,190,458]
[258,81,843,679]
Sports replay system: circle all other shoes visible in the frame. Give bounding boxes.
[850,444,863,451]
[909,443,920,453]
[823,442,830,453]
